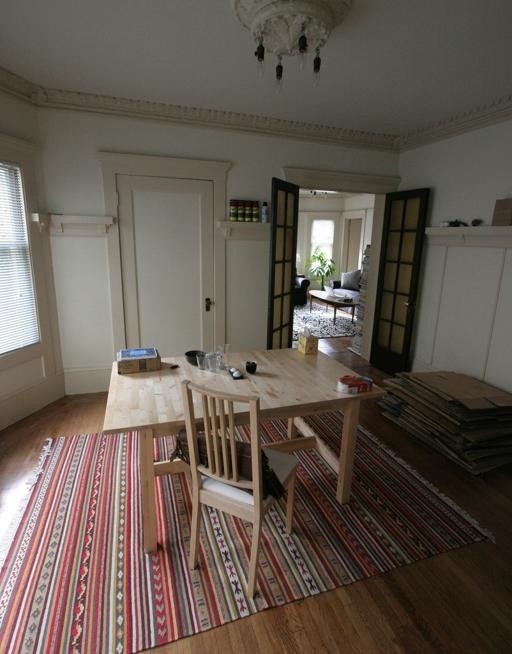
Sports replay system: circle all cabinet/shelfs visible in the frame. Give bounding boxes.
[413,227,512,393]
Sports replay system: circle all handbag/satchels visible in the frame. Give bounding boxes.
[167,430,285,500]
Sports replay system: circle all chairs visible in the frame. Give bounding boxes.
[330,279,360,317]
[180,380,298,601]
[294,274,310,305]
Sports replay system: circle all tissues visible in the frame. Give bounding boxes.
[298,326,318,355]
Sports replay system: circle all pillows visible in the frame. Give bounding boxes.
[340,270,361,291]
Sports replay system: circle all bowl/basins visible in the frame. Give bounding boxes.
[185,350,206,364]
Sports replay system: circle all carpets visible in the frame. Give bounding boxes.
[292,303,359,340]
[0,410,494,654]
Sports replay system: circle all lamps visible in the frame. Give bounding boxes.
[308,191,327,200]
[234,0,355,91]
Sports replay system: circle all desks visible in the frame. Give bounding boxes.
[102,347,387,552]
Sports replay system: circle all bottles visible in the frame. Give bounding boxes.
[228,198,260,223]
[262,201,269,224]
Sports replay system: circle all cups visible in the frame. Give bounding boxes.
[246,361,256,373]
[197,345,230,371]
